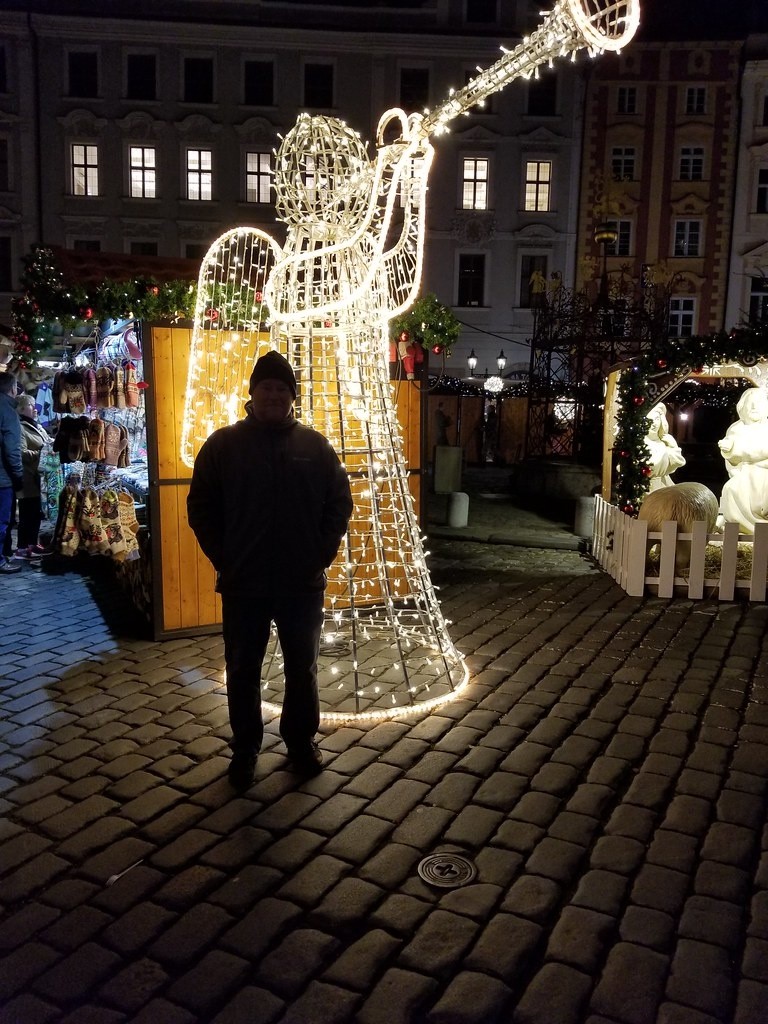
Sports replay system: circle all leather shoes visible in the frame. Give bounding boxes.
[1,562,22,573]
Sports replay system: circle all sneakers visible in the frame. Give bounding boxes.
[15,549,40,560]
[288,734,324,770]
[228,753,258,787]
[30,543,53,557]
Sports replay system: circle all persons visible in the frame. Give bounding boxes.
[0,373,24,572]
[14,395,54,560]
[185,350,356,785]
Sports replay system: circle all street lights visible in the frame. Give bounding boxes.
[467,346,478,376]
[495,347,507,379]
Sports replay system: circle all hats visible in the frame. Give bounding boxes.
[248,349,298,400]
